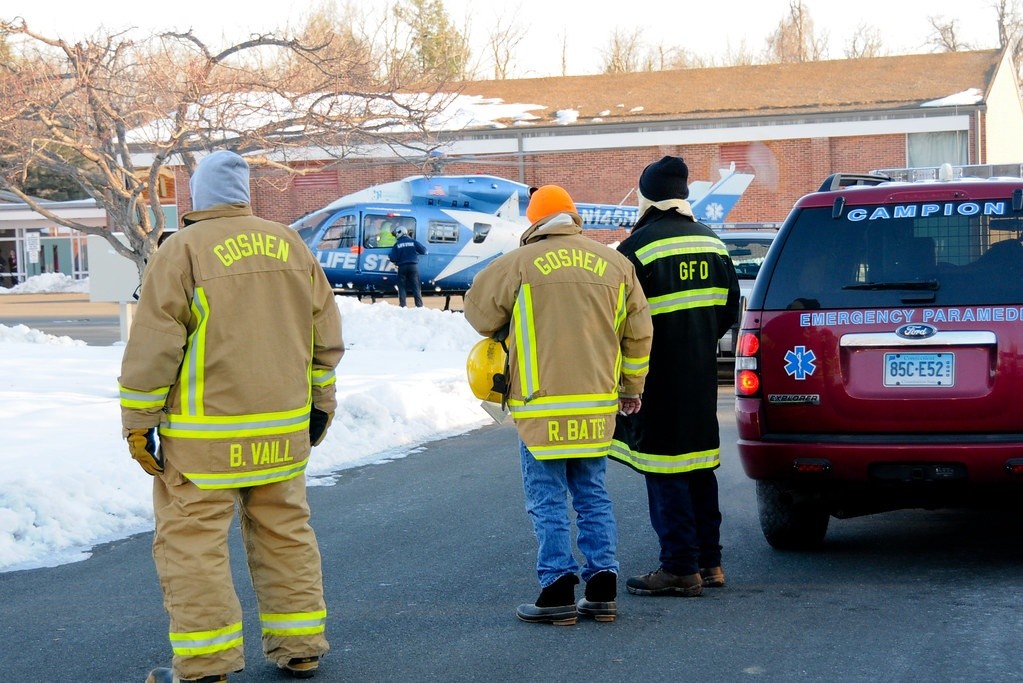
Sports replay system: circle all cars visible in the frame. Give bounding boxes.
[699,218,782,373]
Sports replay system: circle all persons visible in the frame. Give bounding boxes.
[609,156,741,597]
[118,150,344,683]
[8,249,18,285]
[40,246,59,273]
[388,226,427,308]
[0,250,7,286]
[377,216,399,248]
[75,253,79,278]
[465,186,653,625]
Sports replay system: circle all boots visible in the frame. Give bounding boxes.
[515,573,618,626]
[286,656,320,678]
[145,667,230,683]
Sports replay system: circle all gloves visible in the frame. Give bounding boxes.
[126,428,164,476]
[311,404,335,449]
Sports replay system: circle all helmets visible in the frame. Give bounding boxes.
[467,337,517,403]
[395,226,407,239]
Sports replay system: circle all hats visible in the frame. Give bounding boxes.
[638,155,689,202]
[525,184,578,225]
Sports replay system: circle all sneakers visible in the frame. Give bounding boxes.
[626,568,725,597]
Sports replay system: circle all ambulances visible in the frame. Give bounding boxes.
[732,161,1023,552]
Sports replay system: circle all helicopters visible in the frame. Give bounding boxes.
[284,147,757,311]
